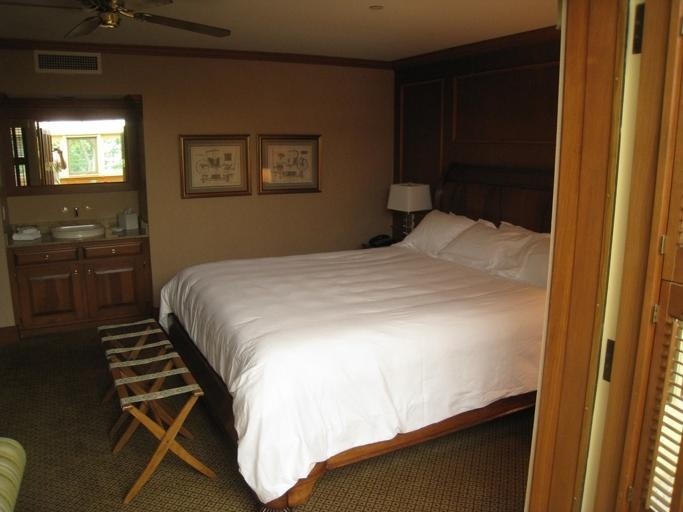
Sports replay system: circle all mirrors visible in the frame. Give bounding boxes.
[1,95,137,197]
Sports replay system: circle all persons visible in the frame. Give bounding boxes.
[52,147,67,184]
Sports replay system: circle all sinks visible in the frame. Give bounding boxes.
[50,222,106,241]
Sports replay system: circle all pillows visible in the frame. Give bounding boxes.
[392,208,552,289]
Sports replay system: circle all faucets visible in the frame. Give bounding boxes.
[70,208,82,220]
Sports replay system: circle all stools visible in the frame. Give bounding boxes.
[93,318,214,504]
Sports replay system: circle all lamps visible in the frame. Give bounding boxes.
[384,184,434,221]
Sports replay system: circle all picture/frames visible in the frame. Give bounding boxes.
[177,133,323,195]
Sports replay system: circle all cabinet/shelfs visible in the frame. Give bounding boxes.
[7,237,152,340]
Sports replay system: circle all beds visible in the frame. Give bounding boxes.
[159,163,557,507]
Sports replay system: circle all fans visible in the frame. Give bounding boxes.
[1,1,231,41]
